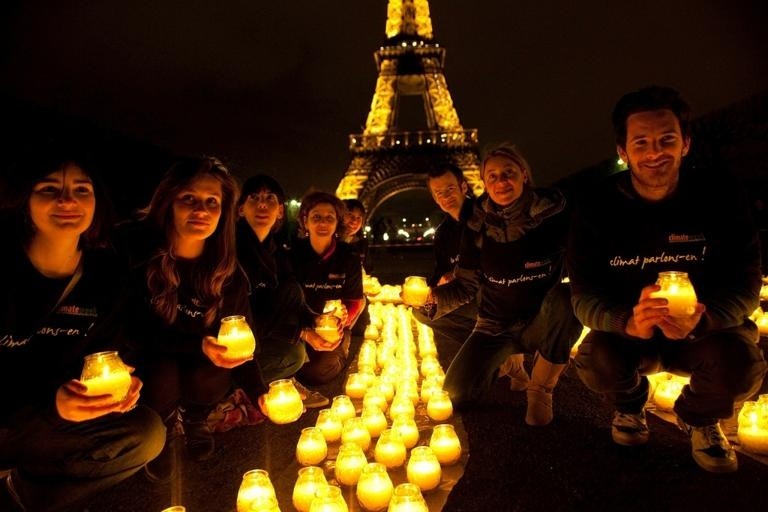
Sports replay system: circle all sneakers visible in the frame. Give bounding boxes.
[291,377,329,407]
[679,412,740,475]
[610,406,651,447]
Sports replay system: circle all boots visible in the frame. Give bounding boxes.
[524,349,566,426]
[500,353,529,392]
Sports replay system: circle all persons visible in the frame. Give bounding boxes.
[561,86,768,475]
[230,172,348,408]
[1,143,170,508]
[100,151,276,486]
[400,142,585,426]
[280,190,367,385]
[421,161,478,345]
[337,198,367,255]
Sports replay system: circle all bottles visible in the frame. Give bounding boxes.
[216,315,256,361]
[404,276,426,309]
[292,297,462,512]
[238,468,281,510]
[654,268,695,320]
[266,378,306,424]
[79,350,132,401]
[649,373,768,454]
